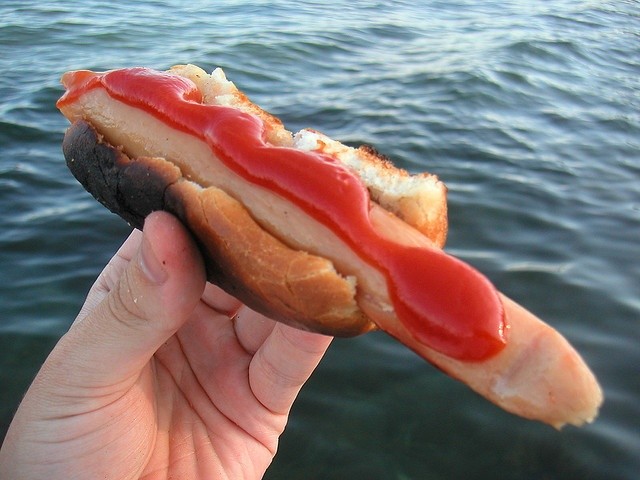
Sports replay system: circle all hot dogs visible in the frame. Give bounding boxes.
[50,62,606,434]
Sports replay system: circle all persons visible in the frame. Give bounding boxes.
[0,210,334,479]
